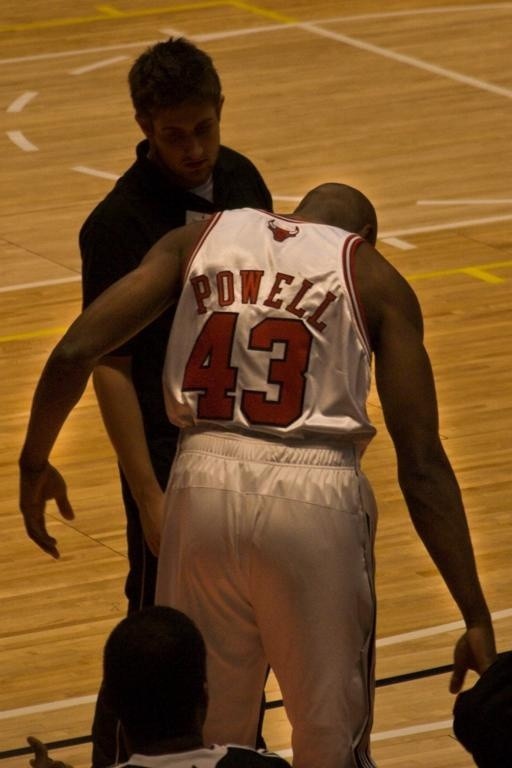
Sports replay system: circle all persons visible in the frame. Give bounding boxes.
[76,35,275,618]
[16,181,497,767]
[22,605,293,767]
